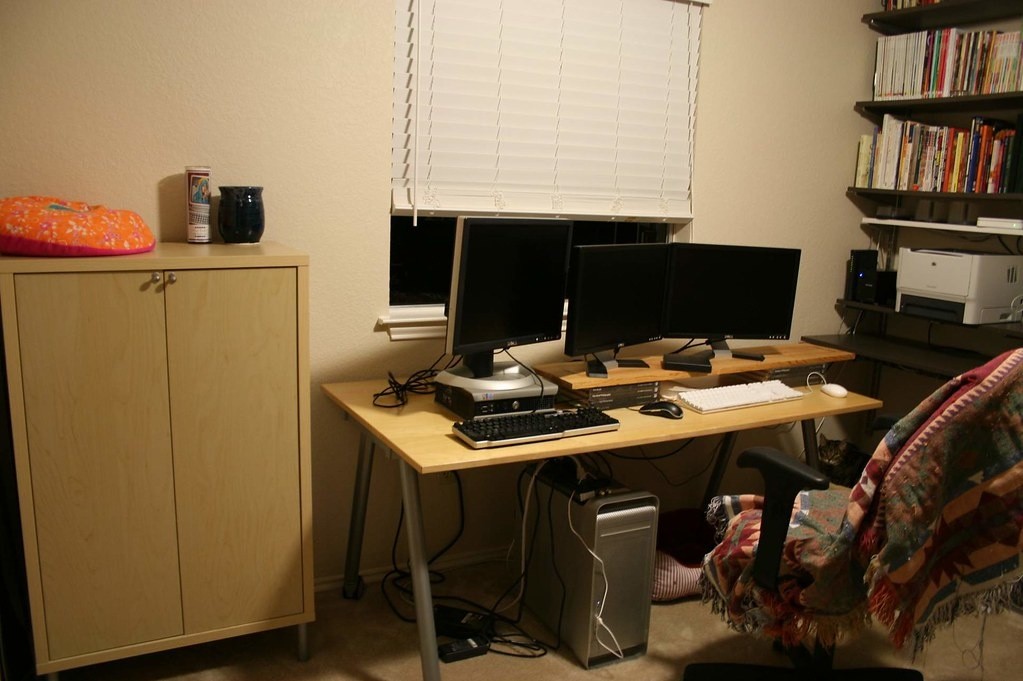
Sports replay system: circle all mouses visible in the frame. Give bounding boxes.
[639,401,685,418]
[821,383,848,398]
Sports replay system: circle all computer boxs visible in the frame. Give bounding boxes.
[434,371,558,421]
[518,468,660,669]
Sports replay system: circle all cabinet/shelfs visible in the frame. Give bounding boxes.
[0,241,315,681]
[848,0,1023,201]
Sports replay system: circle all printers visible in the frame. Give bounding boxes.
[895,248,1023,325]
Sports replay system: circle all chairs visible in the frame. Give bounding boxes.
[683,347,1023,680]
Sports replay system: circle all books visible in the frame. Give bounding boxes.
[884,0,940,12]
[872,28,1023,101]
[855,114,1023,193]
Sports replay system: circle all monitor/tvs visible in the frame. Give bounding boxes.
[564,242,674,378]
[431,217,574,390]
[662,243,802,361]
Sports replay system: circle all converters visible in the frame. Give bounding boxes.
[439,638,490,663]
[432,605,498,641]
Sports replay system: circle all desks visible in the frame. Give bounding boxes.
[321,343,883,681]
[801,332,992,434]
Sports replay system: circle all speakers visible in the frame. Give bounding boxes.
[850,249,878,304]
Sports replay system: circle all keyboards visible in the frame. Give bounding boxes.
[677,380,804,415]
[451,407,621,449]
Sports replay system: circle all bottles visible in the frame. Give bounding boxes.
[218,184,265,246]
